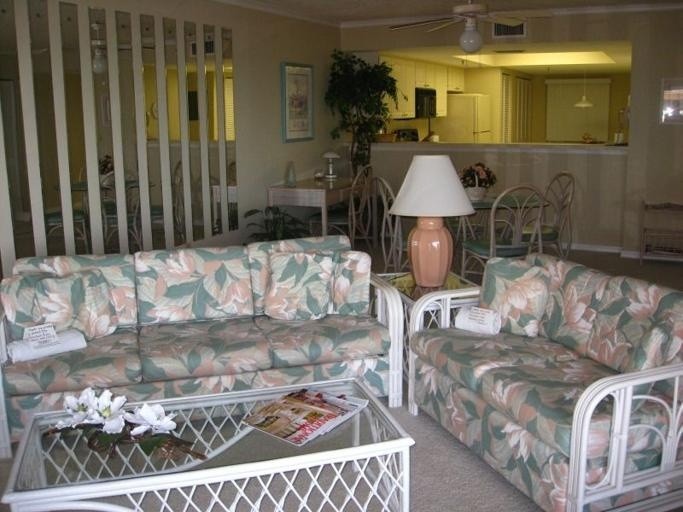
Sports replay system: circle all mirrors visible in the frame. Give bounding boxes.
[660,78,683,126]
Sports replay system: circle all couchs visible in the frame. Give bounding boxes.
[409,253,683,511]
[0,234,405,460]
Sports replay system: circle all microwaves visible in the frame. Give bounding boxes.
[415,88,436,119]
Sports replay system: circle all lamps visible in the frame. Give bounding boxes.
[387,155,475,288]
[574,67,594,108]
[459,18,483,53]
[320,151,341,177]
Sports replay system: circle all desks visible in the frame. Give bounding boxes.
[269,173,380,250]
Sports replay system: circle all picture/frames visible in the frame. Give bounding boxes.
[281,62,316,143]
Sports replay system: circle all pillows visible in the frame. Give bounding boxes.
[0,270,119,341]
[482,257,546,338]
[266,252,371,322]
[625,317,674,413]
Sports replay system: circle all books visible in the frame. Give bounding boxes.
[242,388,369,446]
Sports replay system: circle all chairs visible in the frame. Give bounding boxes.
[34,155,238,255]
[307,165,575,287]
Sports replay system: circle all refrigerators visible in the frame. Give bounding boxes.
[429,93,491,145]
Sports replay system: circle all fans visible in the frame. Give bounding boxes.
[388,0,552,35]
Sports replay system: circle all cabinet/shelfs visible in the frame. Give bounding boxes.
[639,200,683,267]
[355,55,535,144]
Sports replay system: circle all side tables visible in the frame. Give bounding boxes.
[374,272,481,384]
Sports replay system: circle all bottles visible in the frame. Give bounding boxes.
[313,168,325,180]
[286,161,296,187]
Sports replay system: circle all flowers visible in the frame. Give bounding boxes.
[459,162,497,188]
[58,386,208,462]
